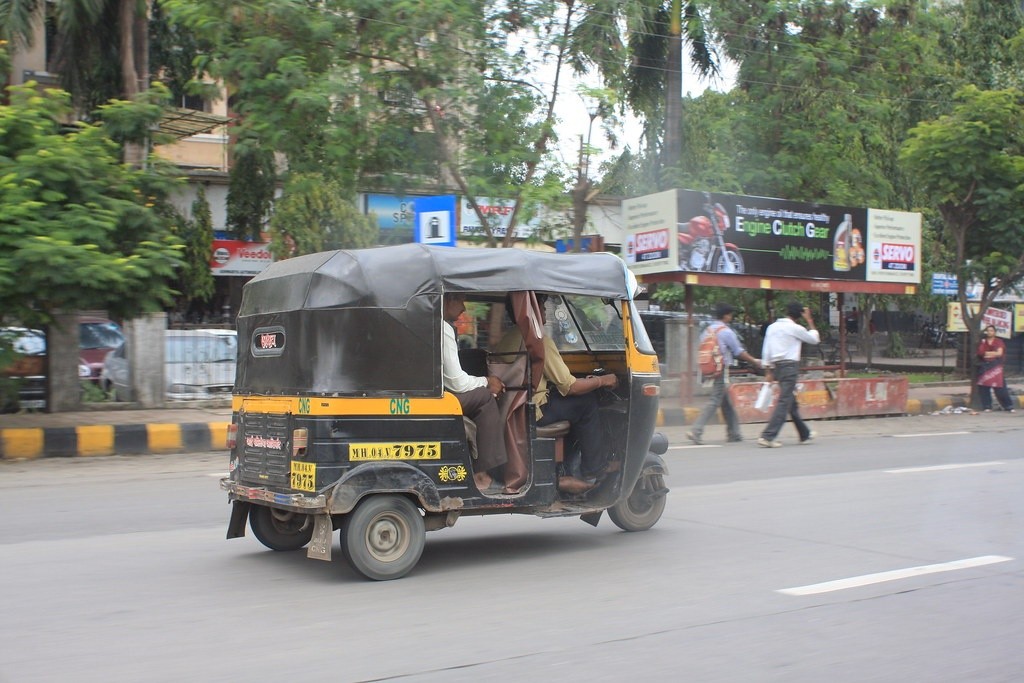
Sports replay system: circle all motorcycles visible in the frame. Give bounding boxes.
[218,242,670,581]
[678,188,745,277]
[917,314,963,349]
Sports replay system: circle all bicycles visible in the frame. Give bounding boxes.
[809,337,853,372]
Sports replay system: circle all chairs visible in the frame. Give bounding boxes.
[458,348,570,439]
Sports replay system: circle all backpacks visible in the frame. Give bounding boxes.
[697,325,728,380]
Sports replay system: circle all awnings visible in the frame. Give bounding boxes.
[152,109,235,144]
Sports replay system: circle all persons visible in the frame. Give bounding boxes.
[976,325,1015,412]
[687,303,821,447]
[442,293,619,495]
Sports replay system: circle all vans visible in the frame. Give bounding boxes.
[75,312,126,386]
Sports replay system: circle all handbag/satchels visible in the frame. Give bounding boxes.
[754,382,772,414]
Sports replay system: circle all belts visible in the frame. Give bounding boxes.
[775,361,800,364]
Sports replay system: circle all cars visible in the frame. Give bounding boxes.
[0,326,50,413]
[99,329,236,407]
[638,310,766,377]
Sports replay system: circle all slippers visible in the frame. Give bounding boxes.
[480,476,506,494]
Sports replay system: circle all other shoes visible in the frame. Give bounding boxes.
[757,437,785,448]
[585,461,621,479]
[984,409,992,412]
[728,435,745,442]
[800,431,818,444]
[686,430,705,445]
[1010,409,1015,412]
